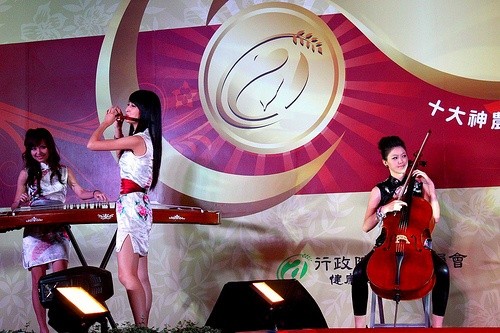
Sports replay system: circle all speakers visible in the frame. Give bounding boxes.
[47,286,109,333]
[206,279,328,333]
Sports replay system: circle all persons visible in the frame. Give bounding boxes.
[86,90,163,329]
[11,127,107,333]
[352,135,450,328]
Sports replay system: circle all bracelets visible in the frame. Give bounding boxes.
[375,206,387,221]
[114,133,124,139]
[93,190,101,195]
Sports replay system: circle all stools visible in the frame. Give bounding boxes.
[370,290,432,329]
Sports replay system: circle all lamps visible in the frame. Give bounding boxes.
[47,286,111,333]
[249,281,284,332]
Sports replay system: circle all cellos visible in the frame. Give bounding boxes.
[366,149,435,326]
